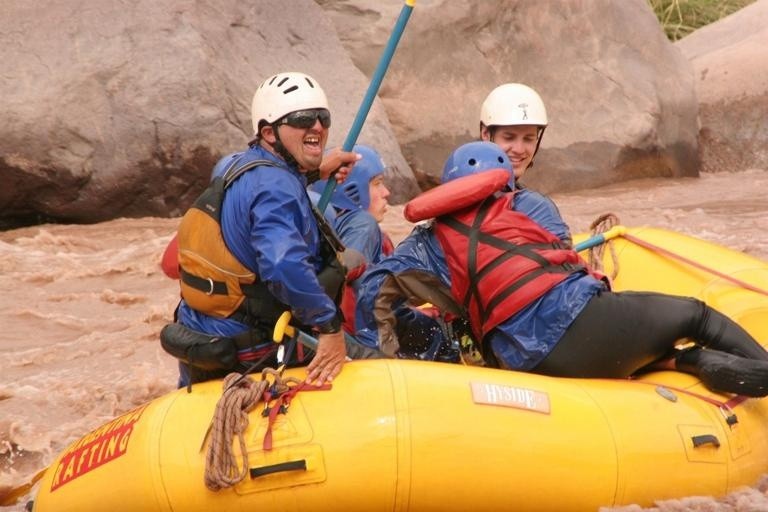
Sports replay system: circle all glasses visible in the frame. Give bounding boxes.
[280,109,332,129]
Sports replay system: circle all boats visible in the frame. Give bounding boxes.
[27,224,766,512]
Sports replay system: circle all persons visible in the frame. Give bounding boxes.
[307,145,394,360]
[441,81,547,365]
[357,140,767,398]
[174,75,362,390]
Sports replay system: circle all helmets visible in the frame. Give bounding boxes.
[250,71,330,136]
[442,140,515,193]
[311,143,386,211]
[479,83,548,128]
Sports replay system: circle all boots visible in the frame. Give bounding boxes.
[674,345,767,399]
